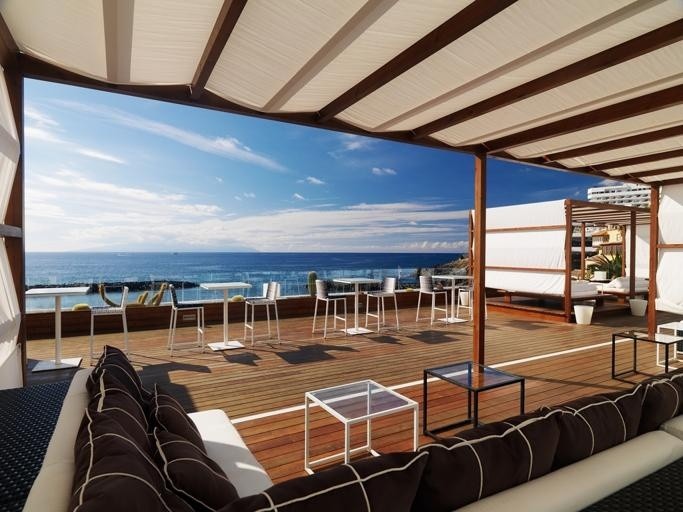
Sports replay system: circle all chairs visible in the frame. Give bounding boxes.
[166,284,204,357]
[89,286,128,366]
[312,279,347,339]
[456,271,488,322]
[243,281,280,347]
[415,276,448,325]
[365,277,398,333]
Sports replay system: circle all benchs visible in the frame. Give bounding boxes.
[21,362,274,512]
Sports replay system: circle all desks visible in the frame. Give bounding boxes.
[430,272,474,325]
[609,321,683,385]
[21,285,89,374]
[302,359,527,476]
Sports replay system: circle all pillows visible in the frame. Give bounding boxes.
[148,380,240,507]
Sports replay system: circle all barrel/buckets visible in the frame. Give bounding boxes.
[574,304,594,326]
[460,291,470,306]
[629,298,648,317]
